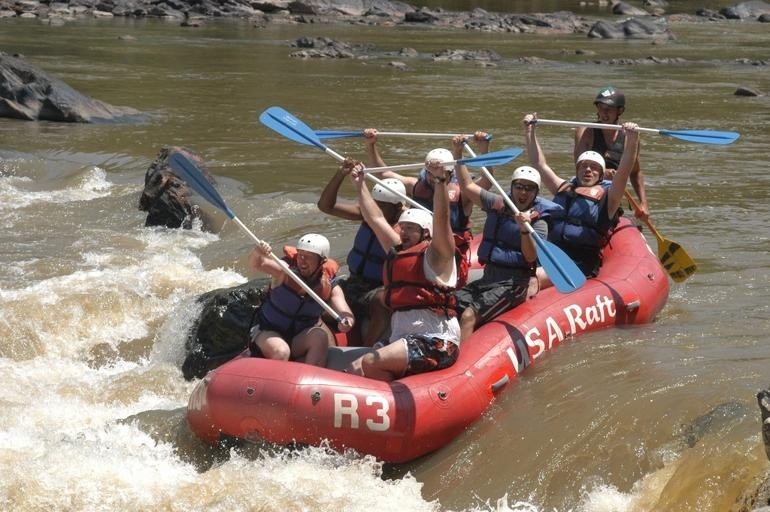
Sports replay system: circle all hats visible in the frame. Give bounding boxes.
[593,88,627,109]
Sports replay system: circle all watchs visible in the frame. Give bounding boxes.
[432,176,447,184]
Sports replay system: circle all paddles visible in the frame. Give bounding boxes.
[168,154,344,326]
[462,140,586,294]
[622,188,695,283]
[525,118,740,146]
[258,105,522,218]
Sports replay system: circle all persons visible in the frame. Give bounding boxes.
[245,232,357,370]
[572,85,651,221]
[361,127,495,243]
[315,155,408,346]
[520,112,641,293]
[350,160,461,384]
[451,134,551,341]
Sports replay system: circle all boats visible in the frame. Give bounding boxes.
[186,210,673,465]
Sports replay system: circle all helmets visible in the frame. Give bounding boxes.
[425,148,456,172]
[576,151,606,175]
[398,209,434,237]
[512,165,541,190]
[295,233,330,260]
[372,178,408,205]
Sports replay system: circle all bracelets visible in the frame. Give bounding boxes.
[521,231,531,235]
